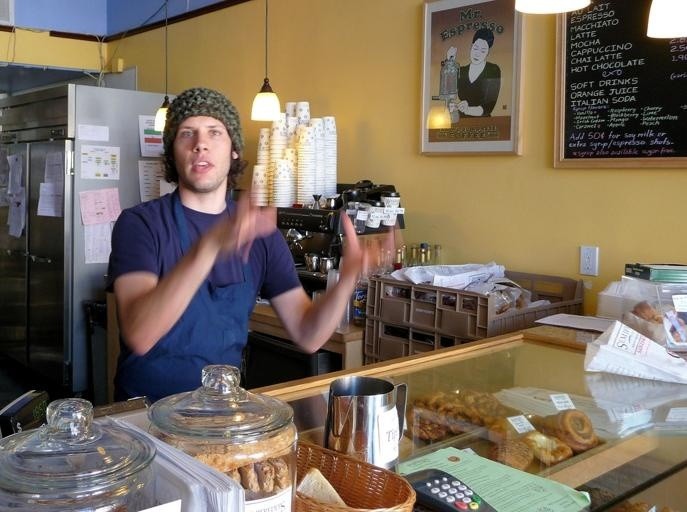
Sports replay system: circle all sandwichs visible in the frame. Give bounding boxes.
[297,467,348,506]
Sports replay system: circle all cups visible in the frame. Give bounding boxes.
[380,191,401,227]
[348,201,369,233]
[286,102,296,116]
[255,128,270,205]
[322,116,337,197]
[322,375,409,475]
[293,204,303,208]
[308,118,325,197]
[269,112,288,201]
[273,159,295,207]
[287,117,298,136]
[365,199,385,228]
[298,127,316,204]
[296,102,310,121]
[305,256,320,272]
[250,165,269,206]
[320,257,334,274]
[304,204,314,209]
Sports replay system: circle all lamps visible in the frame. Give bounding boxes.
[647,0,686,40]
[154,3,171,132]
[251,1,281,122]
[515,1,591,15]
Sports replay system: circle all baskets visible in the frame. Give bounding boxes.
[294,440,416,512]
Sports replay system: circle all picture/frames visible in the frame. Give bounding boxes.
[421,0,521,157]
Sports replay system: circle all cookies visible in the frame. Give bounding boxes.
[226,459,289,501]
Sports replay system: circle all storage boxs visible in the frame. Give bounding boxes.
[365,270,583,366]
[242,332,340,390]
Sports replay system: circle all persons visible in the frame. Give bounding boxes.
[447,28,502,117]
[107,88,370,401]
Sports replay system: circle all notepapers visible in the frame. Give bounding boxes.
[45,153,64,181]
[8,196,25,238]
[80,189,122,225]
[36,181,64,219]
[7,153,23,195]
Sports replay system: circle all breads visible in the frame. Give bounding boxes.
[488,440,534,471]
[404,389,507,439]
[634,300,664,324]
[532,409,598,452]
[488,418,521,443]
[521,430,573,466]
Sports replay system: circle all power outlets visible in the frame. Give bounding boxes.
[579,246,599,276]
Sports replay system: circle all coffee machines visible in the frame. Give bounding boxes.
[432,54,461,124]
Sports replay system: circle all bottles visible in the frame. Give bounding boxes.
[374,241,441,275]
[352,253,371,327]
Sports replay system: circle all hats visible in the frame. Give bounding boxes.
[163,88,244,166]
